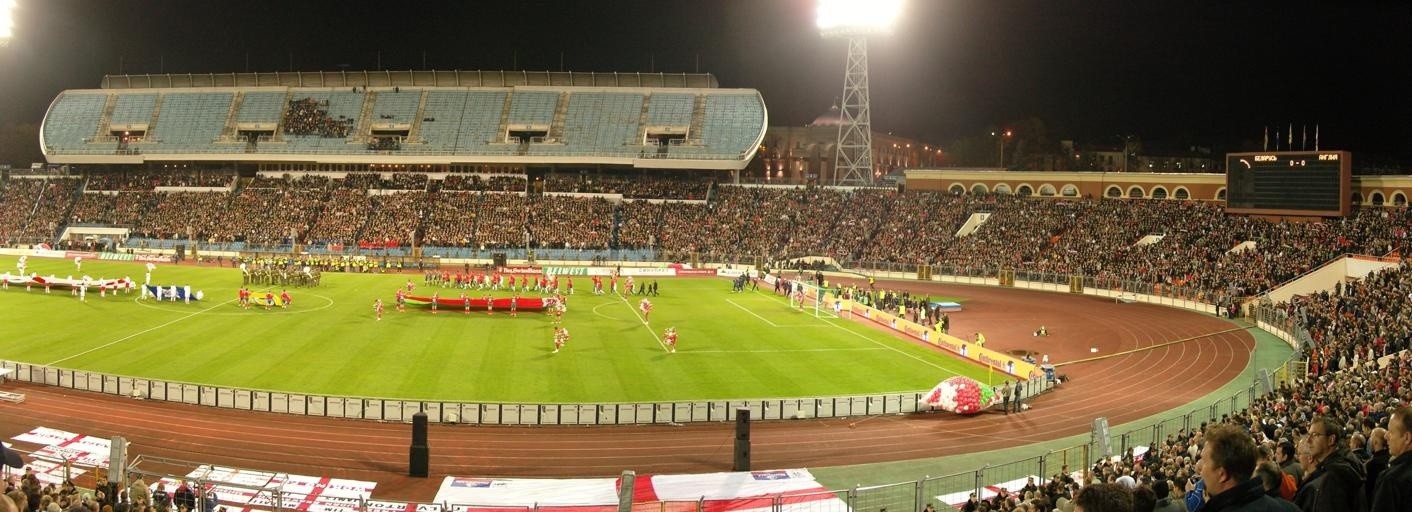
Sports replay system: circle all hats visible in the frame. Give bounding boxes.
[0,442,24,469]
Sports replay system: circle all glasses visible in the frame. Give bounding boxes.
[1307,432,1327,438]
